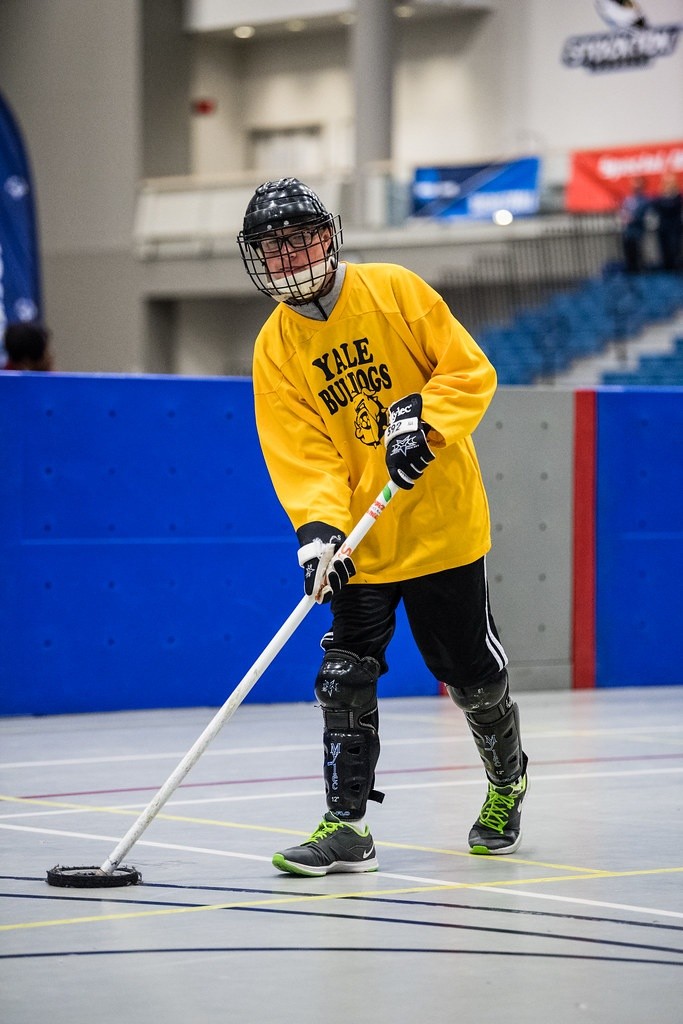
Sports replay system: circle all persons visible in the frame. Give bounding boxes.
[235,178,527,876]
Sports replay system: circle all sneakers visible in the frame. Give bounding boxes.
[274,821,382,879]
[469,763,528,855]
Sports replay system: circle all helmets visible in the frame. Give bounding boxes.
[236,176,347,305]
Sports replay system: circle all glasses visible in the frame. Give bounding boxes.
[259,224,325,253]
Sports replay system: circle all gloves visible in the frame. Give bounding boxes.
[379,391,440,491]
[297,521,355,606]
